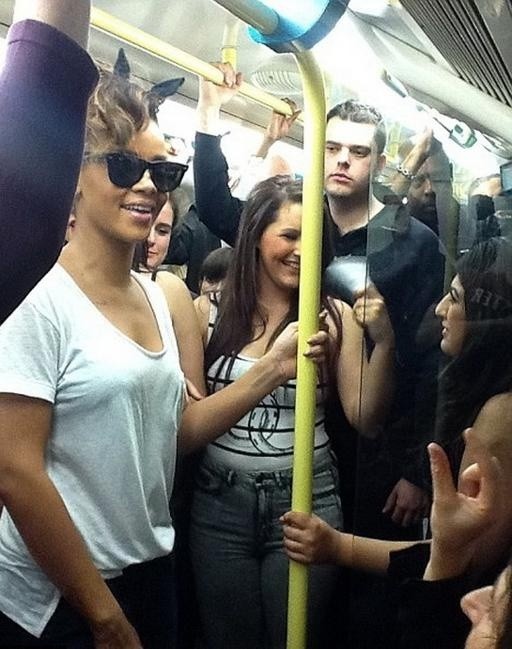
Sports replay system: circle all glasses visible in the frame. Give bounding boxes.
[105,152,189,194]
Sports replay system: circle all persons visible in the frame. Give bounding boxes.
[2,1,511,648]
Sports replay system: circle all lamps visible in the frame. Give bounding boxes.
[252,52,308,96]
